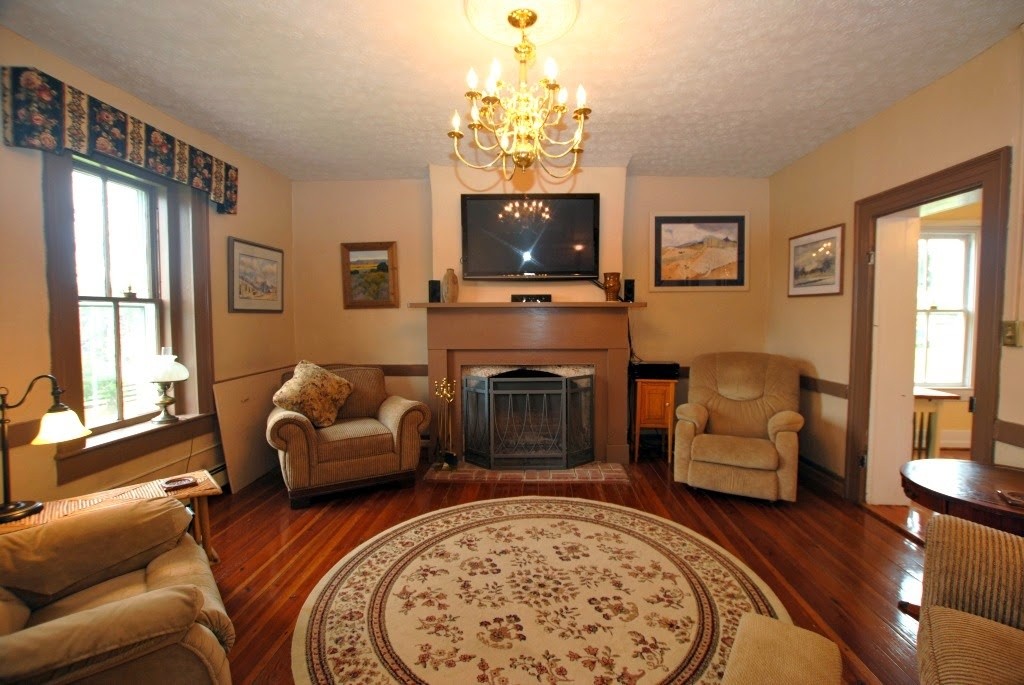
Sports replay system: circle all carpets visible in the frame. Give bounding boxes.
[292,494,794,684]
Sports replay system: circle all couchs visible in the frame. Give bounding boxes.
[0,495,239,684]
[265,364,435,510]
[670,354,805,508]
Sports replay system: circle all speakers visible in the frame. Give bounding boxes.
[429,280,441,303]
[624,279,636,302]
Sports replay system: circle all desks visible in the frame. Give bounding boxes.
[3,467,224,567]
[913,385,959,461]
[634,377,677,461]
[897,456,1024,620]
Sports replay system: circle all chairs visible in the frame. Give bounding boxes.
[911,512,1024,685]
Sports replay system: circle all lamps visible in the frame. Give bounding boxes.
[1,373,94,521]
[148,346,190,423]
[448,0,591,185]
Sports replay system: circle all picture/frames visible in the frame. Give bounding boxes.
[226,235,287,314]
[340,240,402,309]
[645,209,751,294]
[786,222,844,298]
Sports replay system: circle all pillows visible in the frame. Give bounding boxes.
[269,360,356,425]
[725,611,847,684]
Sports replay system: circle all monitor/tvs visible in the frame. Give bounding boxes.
[460,194,600,282]
